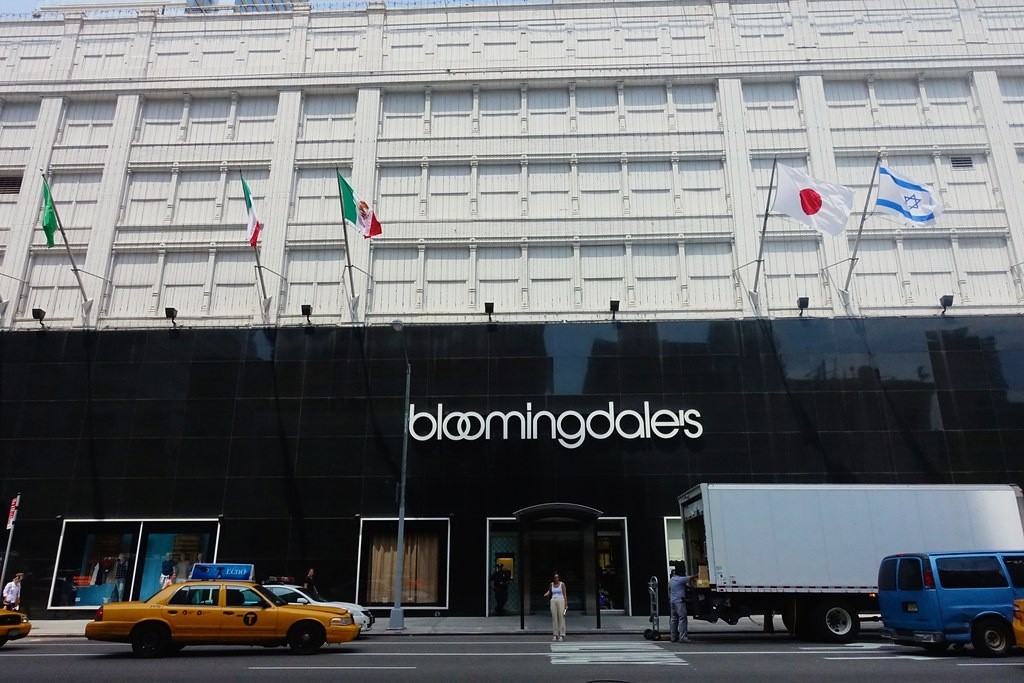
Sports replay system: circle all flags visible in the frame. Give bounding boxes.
[874,164,940,228]
[338,172,382,239]
[772,162,855,236]
[241,177,260,248]
[42,181,58,249]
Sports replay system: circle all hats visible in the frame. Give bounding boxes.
[497,563,504,568]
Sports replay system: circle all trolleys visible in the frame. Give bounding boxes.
[643,575,670,642]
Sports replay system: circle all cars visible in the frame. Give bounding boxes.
[198,576,376,633]
[83,562,362,659]
[0,607,33,649]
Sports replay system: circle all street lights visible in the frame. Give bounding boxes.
[385,319,412,632]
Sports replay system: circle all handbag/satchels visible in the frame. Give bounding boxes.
[13,595,20,607]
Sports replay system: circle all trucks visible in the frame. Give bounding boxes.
[662,482,1024,645]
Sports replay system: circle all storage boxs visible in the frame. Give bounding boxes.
[694,566,709,587]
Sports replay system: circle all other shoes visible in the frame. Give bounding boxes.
[552,636,557,641]
[558,636,564,641]
[672,640,676,642]
[679,639,692,643]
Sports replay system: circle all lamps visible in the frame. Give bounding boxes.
[610,301,619,318]
[797,298,808,315]
[301,305,312,322]
[32,309,46,325]
[485,303,494,321]
[940,295,953,313]
[165,308,177,325]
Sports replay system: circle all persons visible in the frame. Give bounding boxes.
[304,568,318,594]
[159,552,206,584]
[115,553,128,602]
[668,563,696,643]
[162,571,177,590]
[2,573,24,611]
[543,571,568,642]
[490,562,512,614]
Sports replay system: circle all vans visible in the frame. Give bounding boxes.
[876,547,1024,657]
